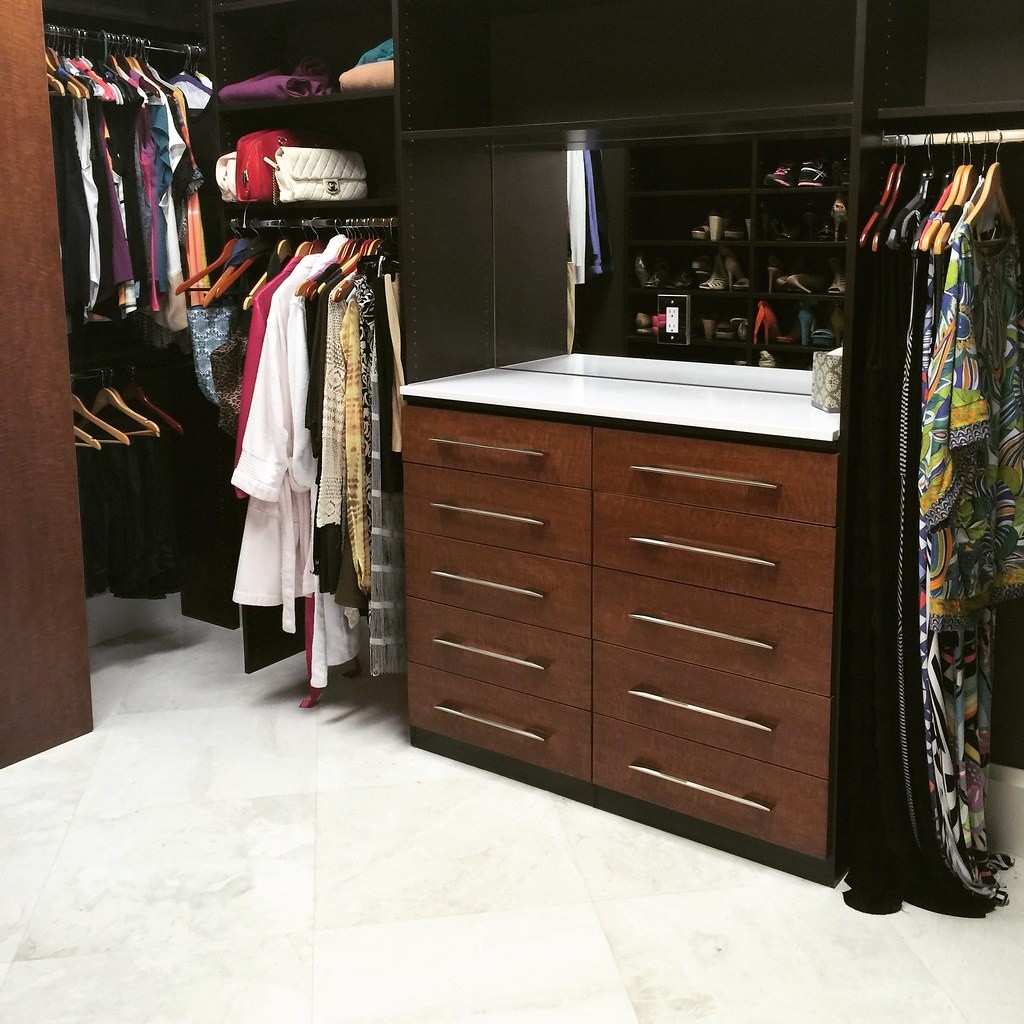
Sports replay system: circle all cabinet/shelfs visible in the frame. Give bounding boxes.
[204,0,401,209]
[397,365,850,890]
[620,126,850,368]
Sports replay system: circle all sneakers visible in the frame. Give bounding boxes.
[764,161,797,187]
[797,160,831,186]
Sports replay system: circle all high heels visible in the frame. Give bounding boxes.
[629,248,847,293]
[690,196,847,242]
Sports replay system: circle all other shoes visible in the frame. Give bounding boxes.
[758,349,778,367]
[635,305,834,346]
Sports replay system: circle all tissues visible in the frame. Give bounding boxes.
[811,347,843,413]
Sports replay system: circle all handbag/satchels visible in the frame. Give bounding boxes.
[215,152,237,202]
[261,147,369,205]
[236,128,317,202]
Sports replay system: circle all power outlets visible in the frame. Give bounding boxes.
[657,295,690,346]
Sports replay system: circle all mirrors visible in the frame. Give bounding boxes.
[491,124,851,396]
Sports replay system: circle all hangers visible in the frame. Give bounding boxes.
[858,130,1005,255]
[44,24,211,110]
[175,217,398,305]
[72,362,183,451]
